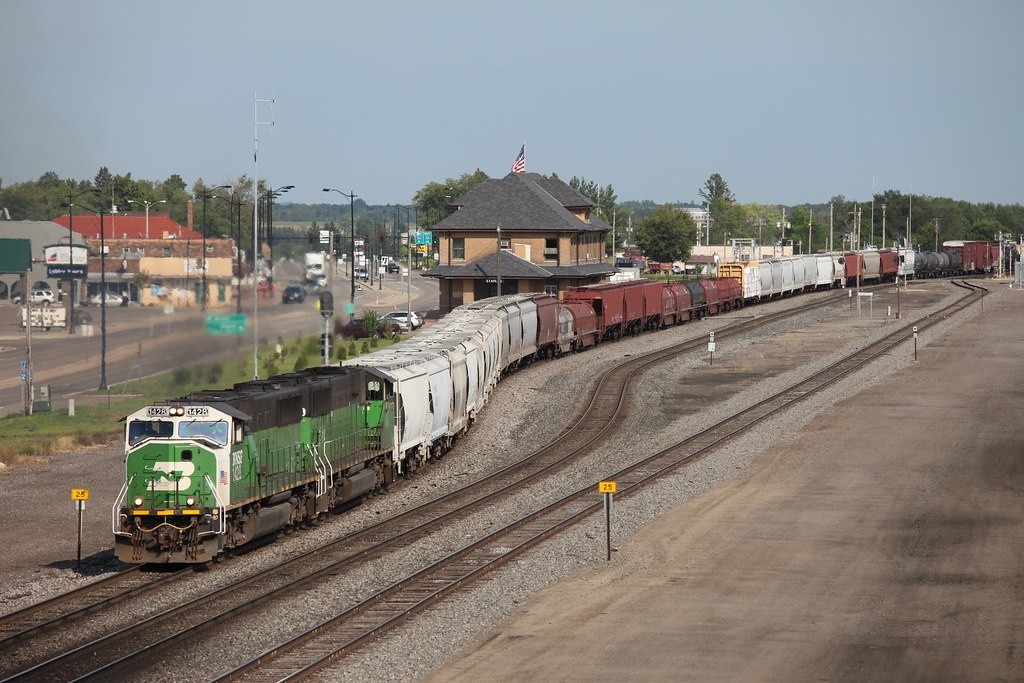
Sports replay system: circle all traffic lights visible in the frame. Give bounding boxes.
[320,292,334,319]
[320,331,326,357]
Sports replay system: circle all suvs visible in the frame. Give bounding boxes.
[380,311,425,330]
[341,318,401,338]
[14,290,55,308]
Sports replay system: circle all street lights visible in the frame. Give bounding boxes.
[201,183,232,311]
[126,197,167,241]
[67,187,103,336]
[213,194,246,317]
[58,204,108,392]
[251,182,294,281]
[322,187,359,323]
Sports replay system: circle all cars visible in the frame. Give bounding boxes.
[80,292,125,309]
[311,275,328,287]
[62,307,92,325]
[281,285,307,305]
[386,321,411,335]
[385,263,399,275]
[353,268,369,281]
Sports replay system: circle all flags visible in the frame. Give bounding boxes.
[511,145,526,175]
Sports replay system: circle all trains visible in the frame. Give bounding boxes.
[109,236,1019,569]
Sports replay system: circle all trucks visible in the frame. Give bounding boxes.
[302,252,326,281]
